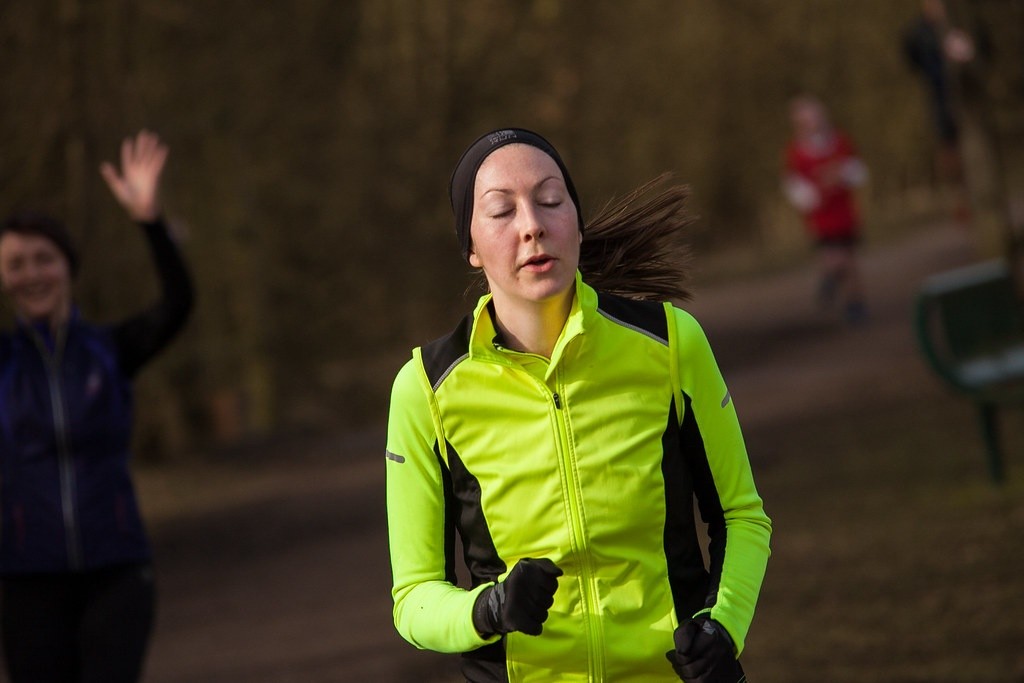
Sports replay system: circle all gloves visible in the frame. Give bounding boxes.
[472,557,564,641]
[666,615,748,683]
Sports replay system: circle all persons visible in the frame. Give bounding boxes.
[897,2,998,190]
[782,93,870,319]
[1,128,195,683]
[386,128,772,683]
